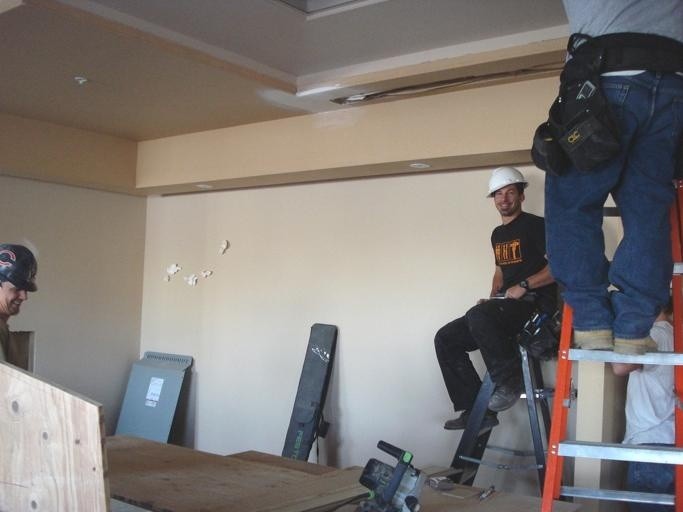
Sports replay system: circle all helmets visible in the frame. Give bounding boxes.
[486,166,530,198]
[0,243,37,291]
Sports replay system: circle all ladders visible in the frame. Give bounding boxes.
[447,343,552,499]
[540,177,683,512]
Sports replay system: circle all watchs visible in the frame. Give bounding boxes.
[519,279,530,291]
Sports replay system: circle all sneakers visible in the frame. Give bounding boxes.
[573,329,614,351]
[487,370,524,412]
[613,334,658,356]
[443,410,499,429]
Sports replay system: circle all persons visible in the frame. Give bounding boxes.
[611,294,677,512]
[544,0,682,354]
[0,243,38,371]
[434,167,561,430]
